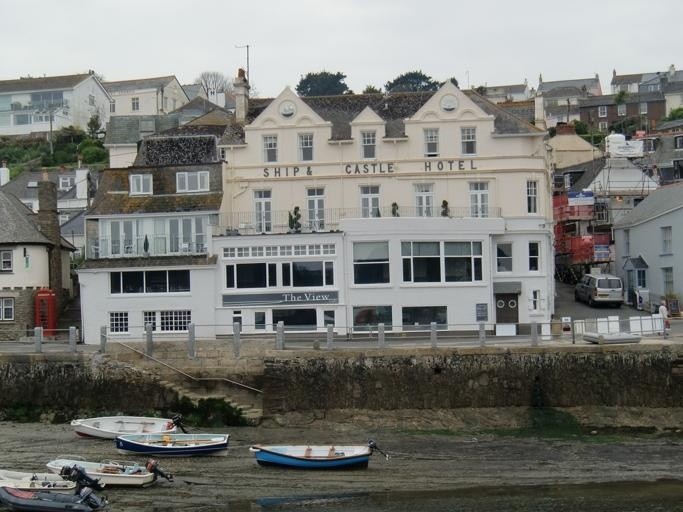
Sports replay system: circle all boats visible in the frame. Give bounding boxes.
[250,444,373,470]
[71,416,230,457]
[0,459,162,512]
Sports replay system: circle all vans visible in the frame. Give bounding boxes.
[574,273,624,308]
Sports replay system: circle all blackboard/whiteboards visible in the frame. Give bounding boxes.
[668,299,680,314]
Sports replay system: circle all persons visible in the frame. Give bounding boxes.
[658,301,670,336]
